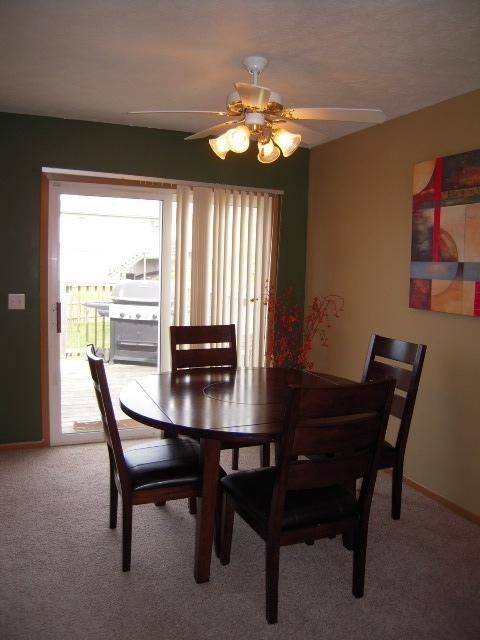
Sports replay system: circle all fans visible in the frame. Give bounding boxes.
[124,53,388,171]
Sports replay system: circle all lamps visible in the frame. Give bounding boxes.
[205,121,301,165]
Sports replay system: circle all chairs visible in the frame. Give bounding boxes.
[87,325,426,624]
